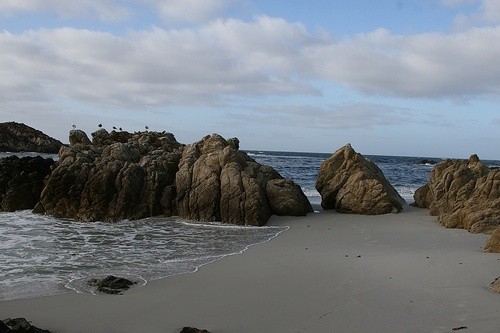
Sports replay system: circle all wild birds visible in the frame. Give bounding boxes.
[113,127,116,129]
[72,125,76,128]
[145,126,149,130]
[119,128,122,131]
[98,123,102,127]
